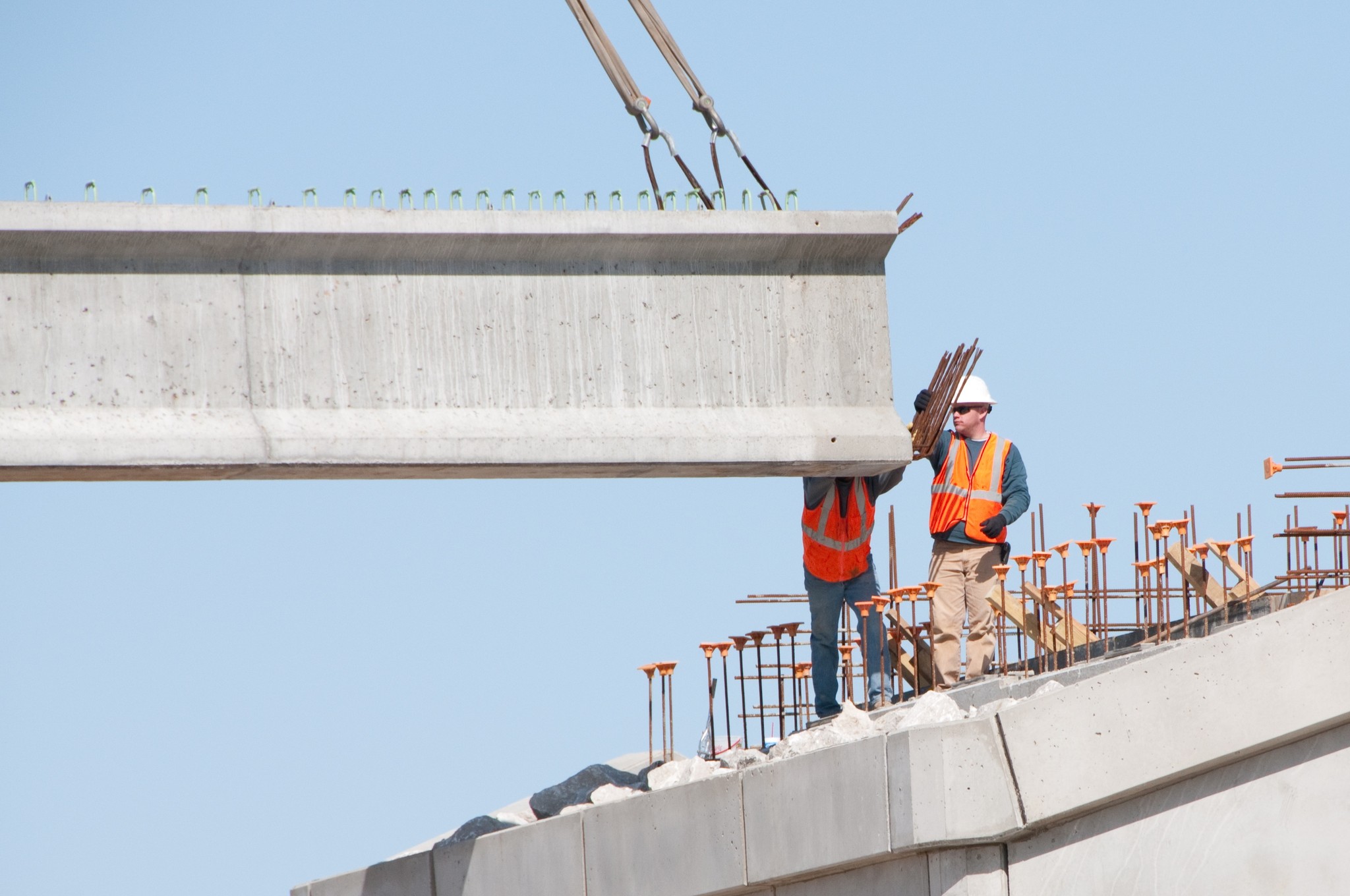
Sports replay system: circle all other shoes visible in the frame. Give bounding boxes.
[872,700,894,710]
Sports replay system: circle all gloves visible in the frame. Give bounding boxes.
[914,389,932,411]
[980,513,1006,538]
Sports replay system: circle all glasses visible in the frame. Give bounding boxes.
[953,406,983,413]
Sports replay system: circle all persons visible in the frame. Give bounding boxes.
[914,375,1030,692]
[803,466,906,718]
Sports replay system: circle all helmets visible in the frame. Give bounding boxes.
[950,375,997,405]
